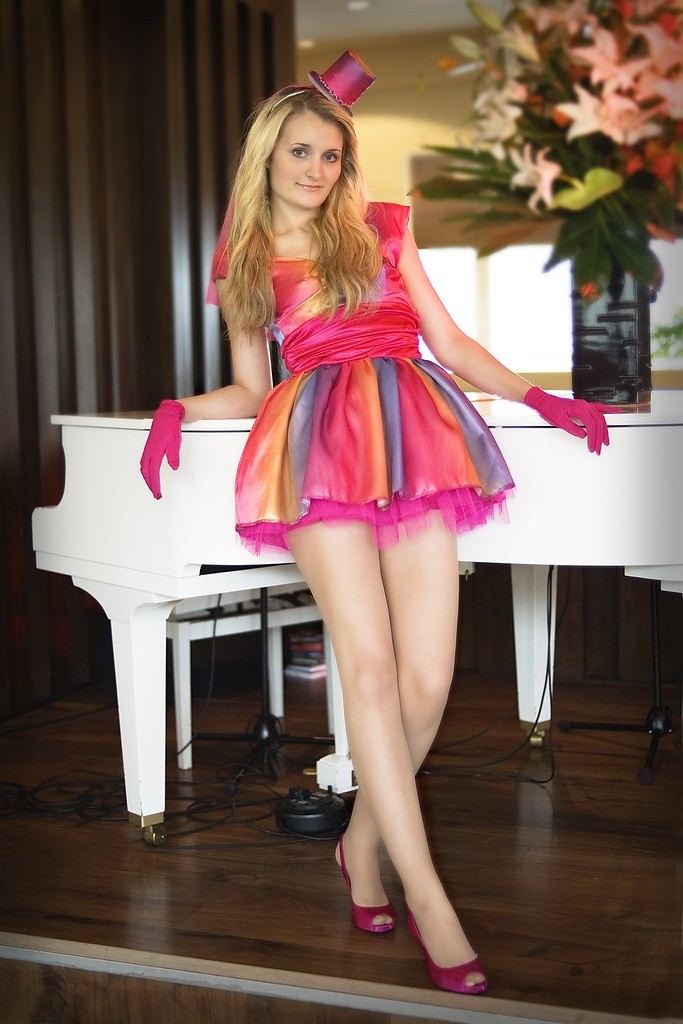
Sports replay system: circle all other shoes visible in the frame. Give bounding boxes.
[404,906,489,995]
[337,828,395,934]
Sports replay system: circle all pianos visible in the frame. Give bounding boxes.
[27,402,683,848]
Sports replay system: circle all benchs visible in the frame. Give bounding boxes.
[165,593,354,771]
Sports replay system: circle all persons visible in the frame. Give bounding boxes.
[139,48,621,992]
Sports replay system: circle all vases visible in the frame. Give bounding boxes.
[572,227,652,414]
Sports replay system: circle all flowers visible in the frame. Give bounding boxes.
[405,0,683,301]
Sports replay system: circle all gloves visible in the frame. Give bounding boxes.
[138,396,187,501]
[523,387,622,456]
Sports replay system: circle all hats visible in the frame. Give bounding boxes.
[306,47,377,120]
